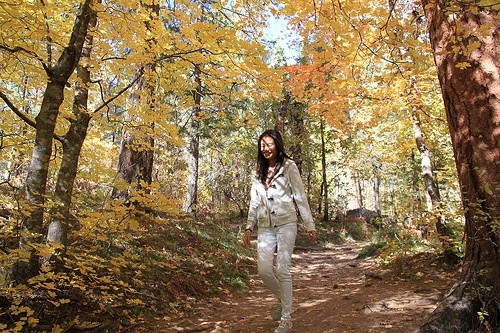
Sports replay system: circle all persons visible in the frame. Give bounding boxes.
[243,128,317,333]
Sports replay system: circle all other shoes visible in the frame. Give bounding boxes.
[273,320,294,333]
[271,297,282,320]
